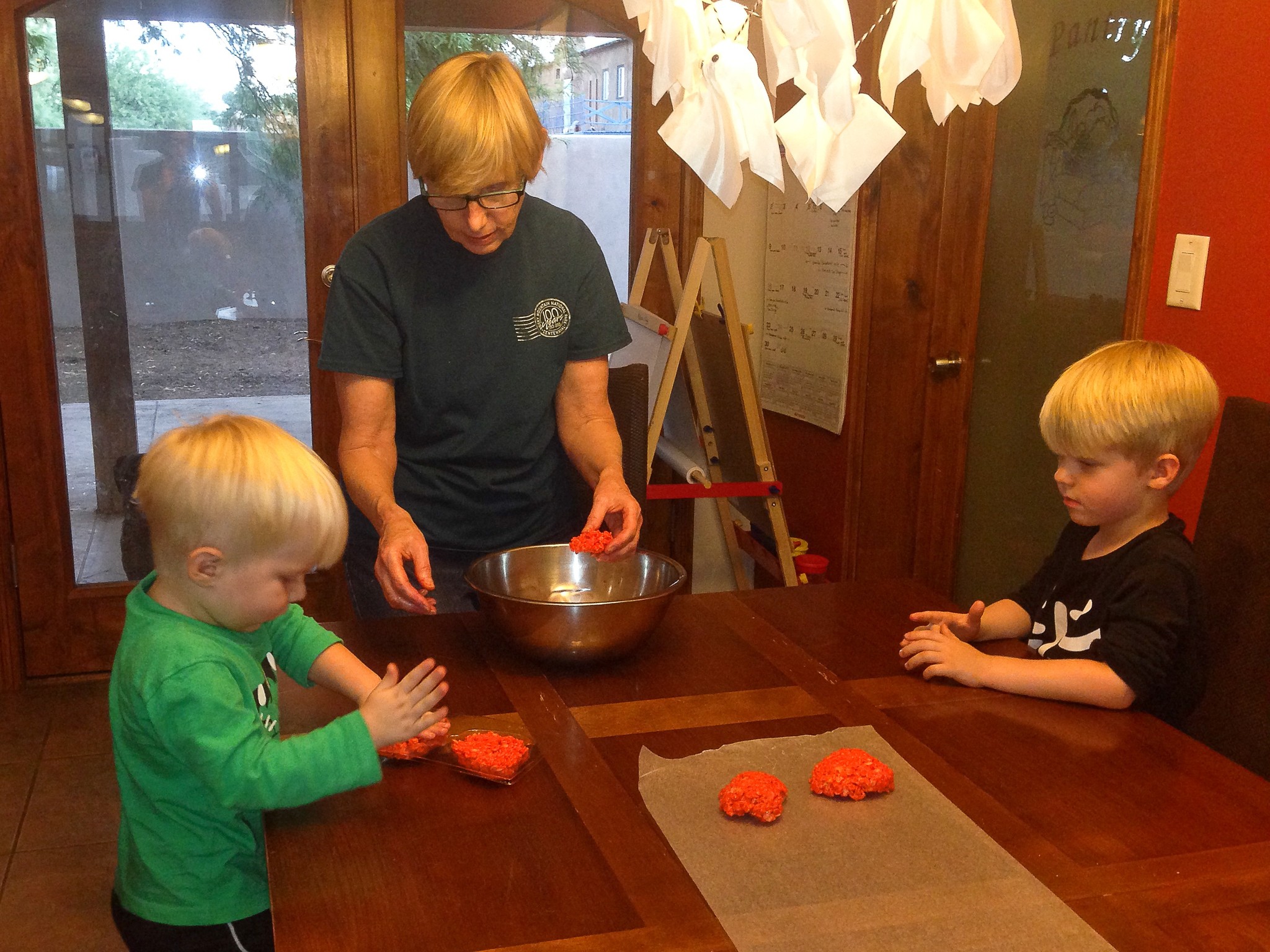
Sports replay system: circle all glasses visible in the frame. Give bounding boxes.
[417,174,529,211]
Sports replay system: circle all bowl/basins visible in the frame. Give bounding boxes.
[464,542,687,664]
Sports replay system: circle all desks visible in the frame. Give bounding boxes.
[264,574,1270,952]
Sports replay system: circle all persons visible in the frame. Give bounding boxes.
[135,113,302,309]
[107,411,448,951]
[327,53,644,622]
[899,340,1220,732]
